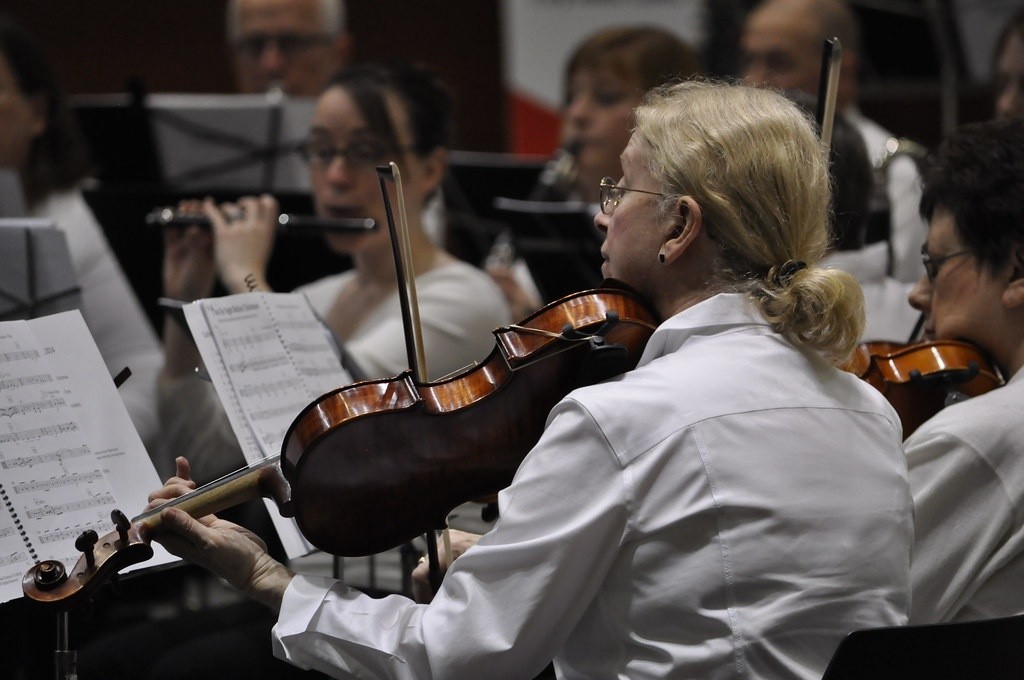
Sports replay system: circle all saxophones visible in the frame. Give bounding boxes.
[473,132,586,280]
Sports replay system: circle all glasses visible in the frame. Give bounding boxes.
[920,242,977,283]
[599,176,704,214]
[230,28,335,58]
[297,137,415,169]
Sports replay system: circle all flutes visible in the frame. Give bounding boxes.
[119,204,384,243]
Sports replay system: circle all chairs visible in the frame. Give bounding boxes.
[821,613,1024,680]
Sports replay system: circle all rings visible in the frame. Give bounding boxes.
[227,210,243,224]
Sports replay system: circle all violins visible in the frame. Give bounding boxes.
[21,279,670,619]
[833,336,1006,437]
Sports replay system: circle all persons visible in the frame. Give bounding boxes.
[2,1,1023,484]
[141,81,915,680]
[860,114,1024,622]
